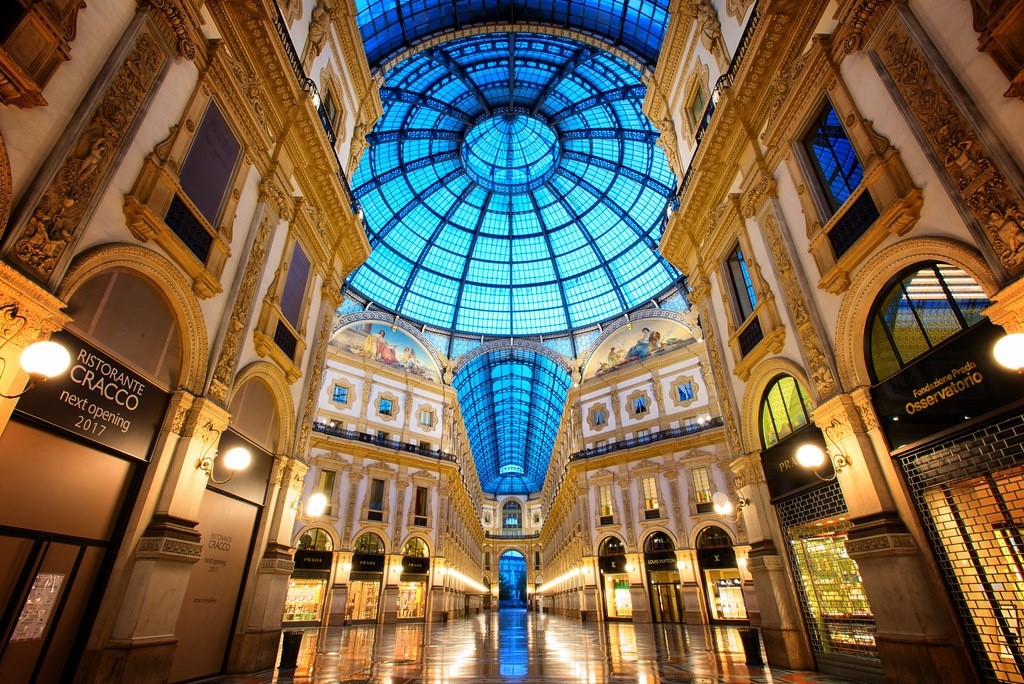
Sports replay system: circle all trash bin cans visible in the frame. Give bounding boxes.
[737,628,763,666]
[279,631,304,668]
[582,612,586,620]
[443,613,448,622]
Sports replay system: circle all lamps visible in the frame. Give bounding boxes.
[195,419,252,484]
[991,333,1024,375]
[0,301,71,399]
[796,419,853,482]
[711,476,751,524]
[288,473,329,527]
